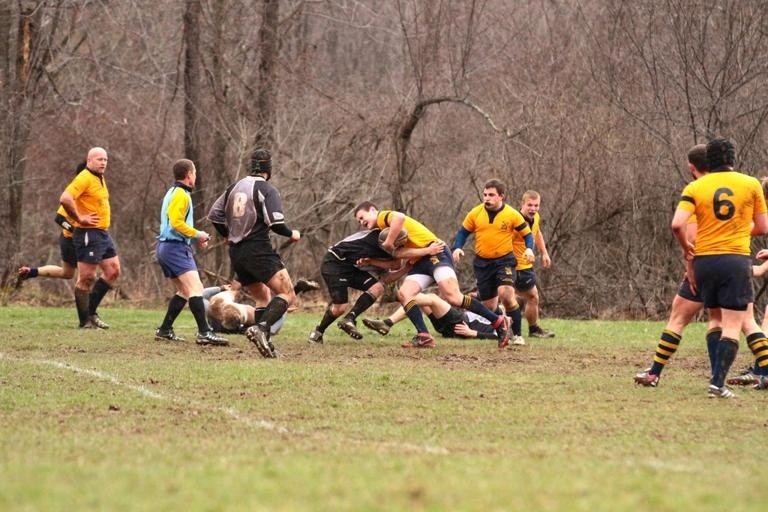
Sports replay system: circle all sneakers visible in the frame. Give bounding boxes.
[194,331,231,348]
[84,315,112,330]
[305,329,325,348]
[705,382,738,402]
[632,367,660,389]
[726,369,760,385]
[336,318,365,340]
[400,332,437,348]
[245,324,277,360]
[155,326,185,343]
[512,329,557,346]
[494,314,513,349]
[362,317,390,336]
[4,265,32,293]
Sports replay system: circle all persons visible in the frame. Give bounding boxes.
[633,135,768,399]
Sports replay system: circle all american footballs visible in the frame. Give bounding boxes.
[378,228,408,247]
